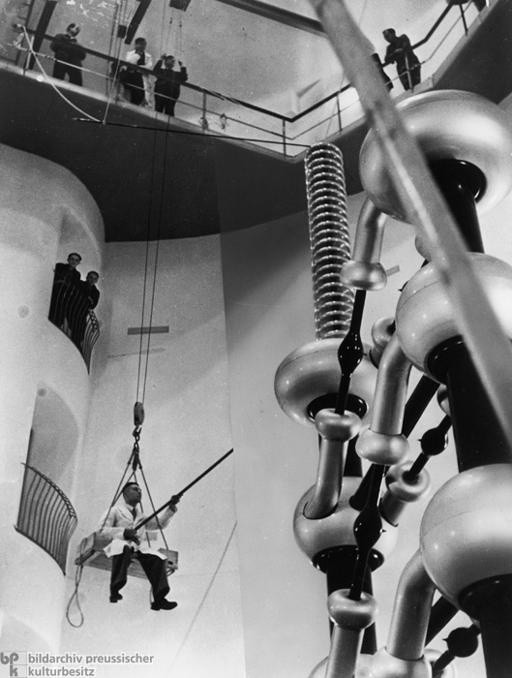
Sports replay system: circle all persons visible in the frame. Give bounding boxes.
[50,23,86,86]
[69,270,100,343]
[100,481,182,611]
[48,252,83,326]
[150,53,188,118]
[124,36,152,103]
[382,28,422,91]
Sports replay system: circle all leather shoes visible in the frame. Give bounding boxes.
[110,592,123,604]
[151,599,177,611]
